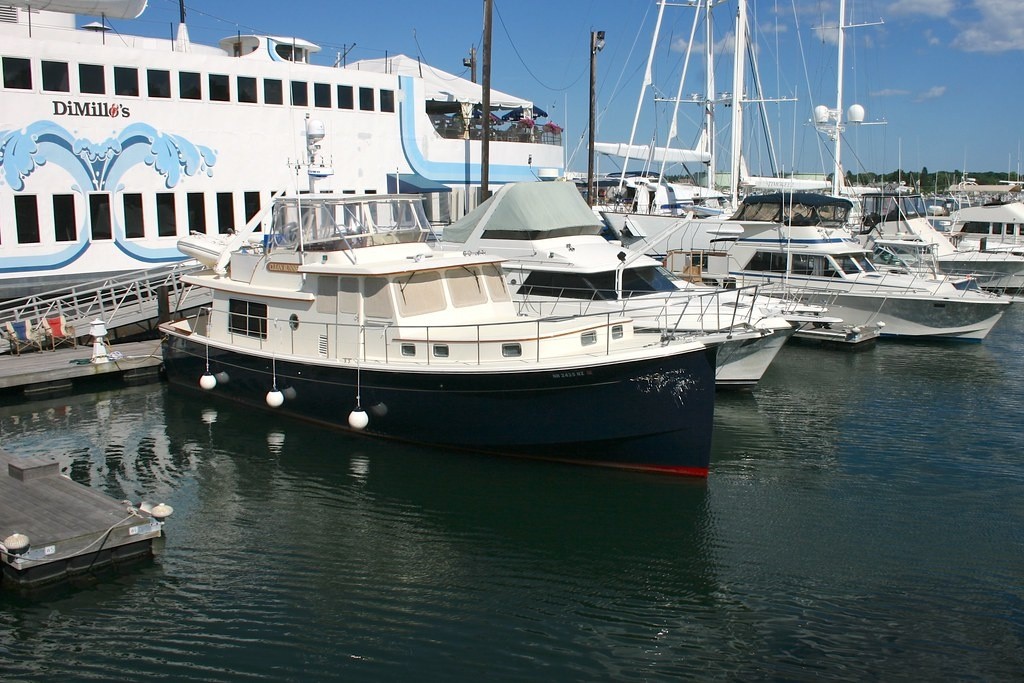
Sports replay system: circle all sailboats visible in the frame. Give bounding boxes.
[157,0,1024,480]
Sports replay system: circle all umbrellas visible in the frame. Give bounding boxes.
[429,114,451,125]
[452,105,501,129]
[502,104,548,133]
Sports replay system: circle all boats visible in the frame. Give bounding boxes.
[0,0,565,301]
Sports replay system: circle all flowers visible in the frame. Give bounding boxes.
[545,122,563,135]
[520,119,534,129]
[489,114,498,123]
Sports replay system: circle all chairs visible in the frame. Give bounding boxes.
[40,315,78,352]
[1,319,44,357]
